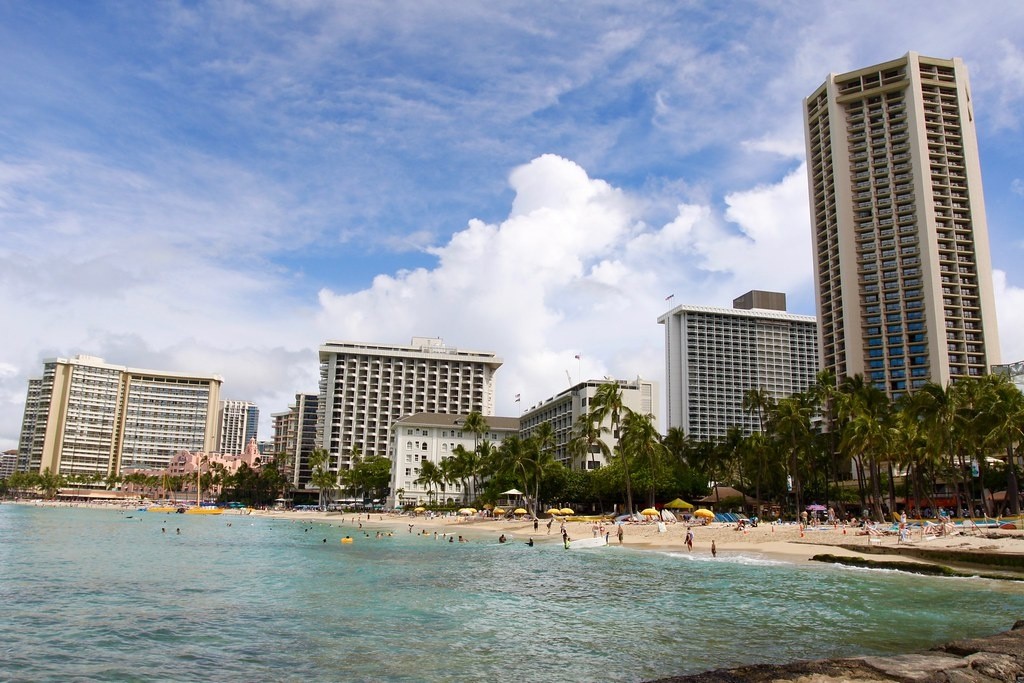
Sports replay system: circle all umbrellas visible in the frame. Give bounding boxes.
[806,505,827,527]
[641,508,660,518]
[694,508,715,520]
[414,504,575,519]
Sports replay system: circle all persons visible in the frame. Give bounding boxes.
[478,508,572,550]
[685,527,693,552]
[161,505,469,543]
[591,504,1013,547]
[711,540,717,557]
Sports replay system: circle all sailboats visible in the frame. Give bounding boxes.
[137,469,224,516]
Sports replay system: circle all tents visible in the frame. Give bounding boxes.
[664,498,694,514]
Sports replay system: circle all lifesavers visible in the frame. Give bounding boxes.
[341,538,354,542]
